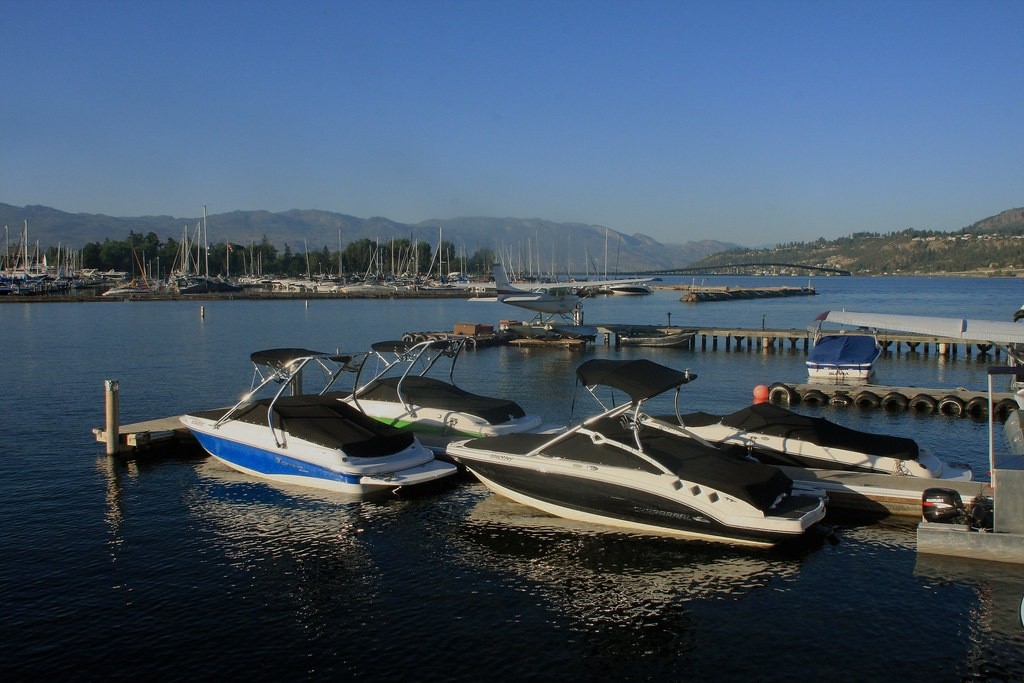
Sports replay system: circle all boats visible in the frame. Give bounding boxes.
[609,283,653,294]
[179,348,459,494]
[0,204,662,298]
[330,338,543,452]
[805,319,884,381]
[444,357,831,551]
[646,402,976,488]
[617,329,700,348]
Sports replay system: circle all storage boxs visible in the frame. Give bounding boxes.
[454,322,495,334]
[499,320,523,327]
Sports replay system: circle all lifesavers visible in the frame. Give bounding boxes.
[733,328,745,340]
[427,336,437,341]
[787,328,800,342]
[769,382,792,403]
[801,390,825,402]
[829,394,852,403]
[854,391,879,405]
[909,393,936,410]
[401,334,413,342]
[906,341,921,348]
[881,392,907,406]
[878,340,893,347]
[965,397,988,410]
[938,395,964,413]
[994,398,1019,412]
[762,328,777,344]
[976,344,993,351]
[414,335,425,342]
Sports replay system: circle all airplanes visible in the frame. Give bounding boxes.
[467,263,599,341]
